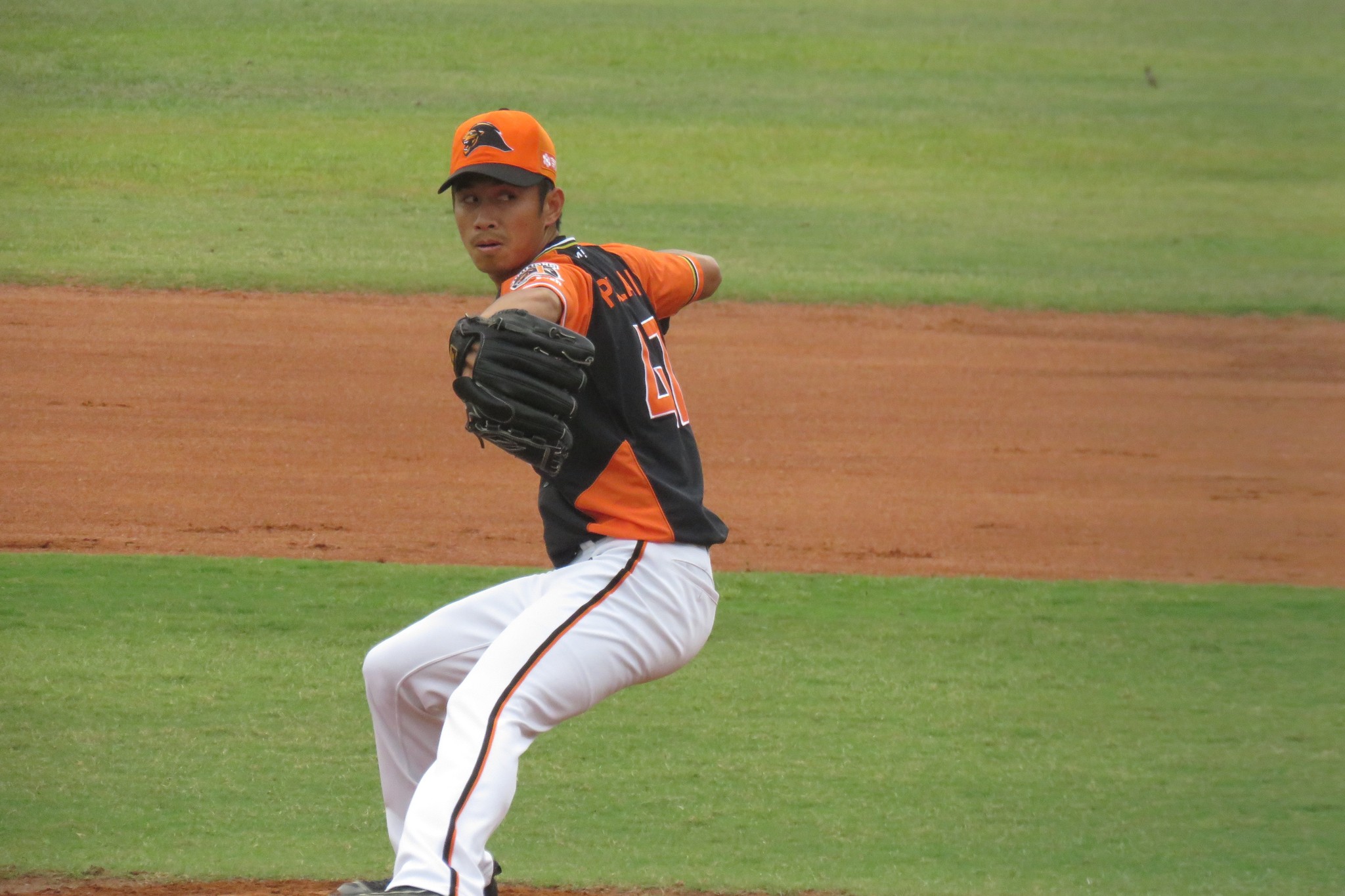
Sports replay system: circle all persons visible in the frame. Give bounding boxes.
[337,107,729,896]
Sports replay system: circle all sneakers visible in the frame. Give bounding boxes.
[360,861,502,896]
[337,877,393,895]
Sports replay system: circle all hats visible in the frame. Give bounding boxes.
[438,107,558,190]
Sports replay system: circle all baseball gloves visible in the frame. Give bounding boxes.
[449,309,595,475]
